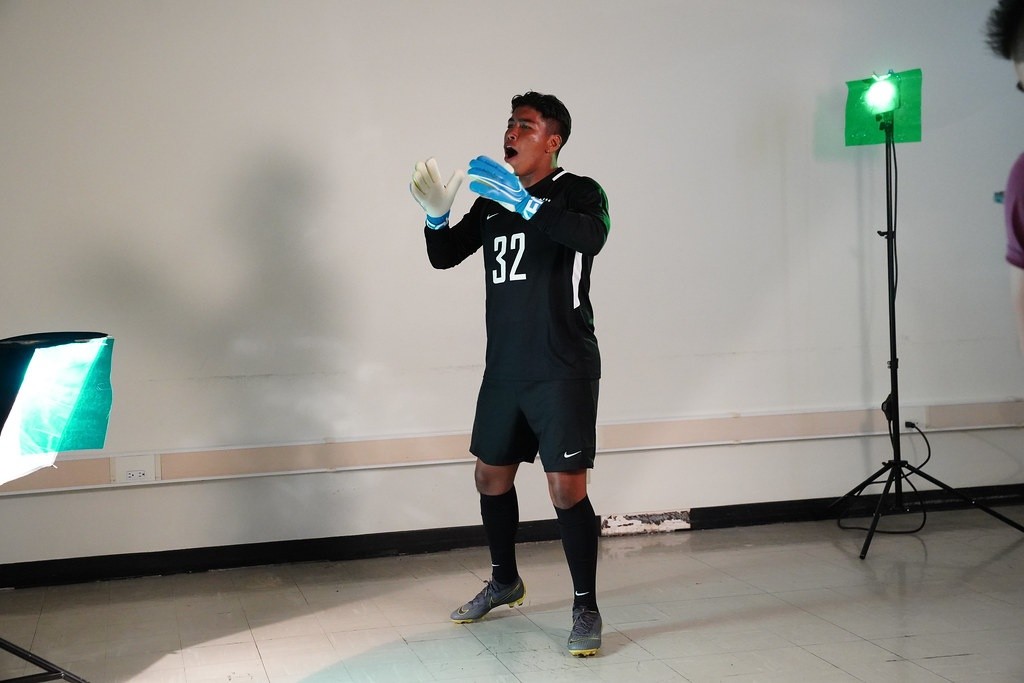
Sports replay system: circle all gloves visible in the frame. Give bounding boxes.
[410,157,464,229]
[468,156,545,221]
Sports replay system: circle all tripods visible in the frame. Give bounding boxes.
[820,109,1024,562]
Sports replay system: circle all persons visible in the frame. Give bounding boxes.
[408,91,610,656]
[984,1,1024,360]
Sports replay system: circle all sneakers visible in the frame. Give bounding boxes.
[450,575,526,624]
[568,605,603,657]
